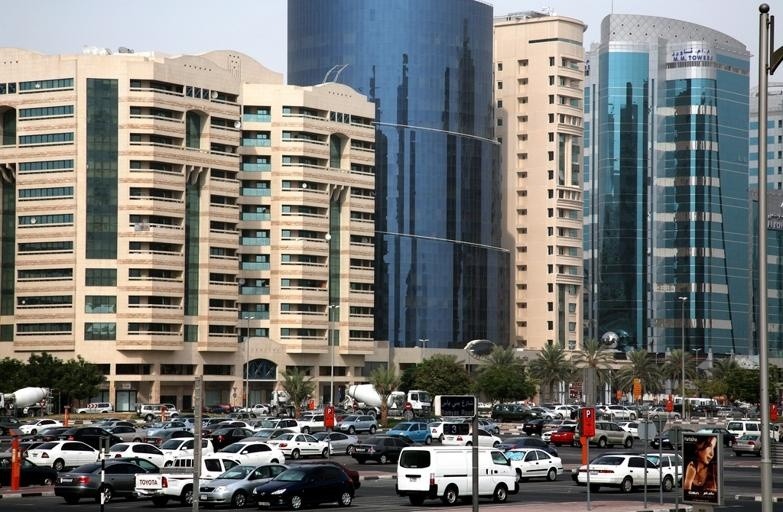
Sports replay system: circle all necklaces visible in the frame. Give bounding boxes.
[694,464,706,484]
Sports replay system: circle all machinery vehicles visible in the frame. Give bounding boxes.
[0,384,54,418]
[344,382,431,419]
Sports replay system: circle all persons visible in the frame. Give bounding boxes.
[159,402,169,422]
[686,437,718,503]
[523,395,533,407]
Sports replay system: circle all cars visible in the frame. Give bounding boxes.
[638,451,684,487]
[578,455,675,494]
[51,459,155,505]
[571,451,645,487]
[249,463,357,510]
[0,393,781,488]
[200,462,289,508]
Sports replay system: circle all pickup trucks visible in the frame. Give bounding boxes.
[137,456,244,505]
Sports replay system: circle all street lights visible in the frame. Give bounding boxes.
[417,337,429,359]
[326,303,342,406]
[677,295,691,420]
[243,316,256,411]
[692,348,702,390]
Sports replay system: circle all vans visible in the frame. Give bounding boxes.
[395,444,523,505]
